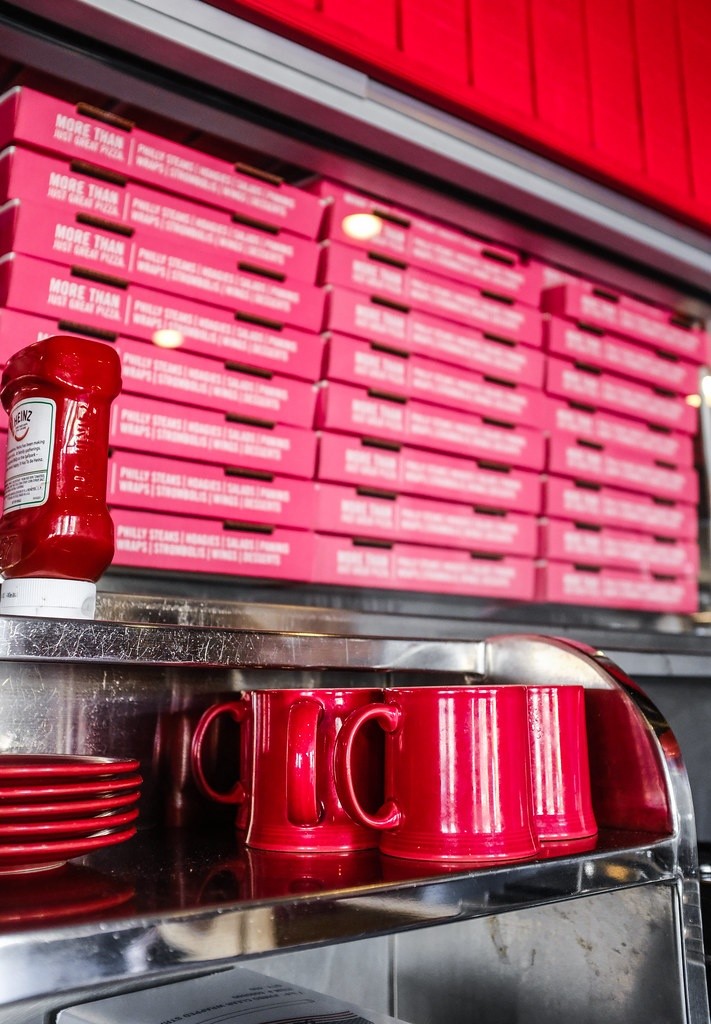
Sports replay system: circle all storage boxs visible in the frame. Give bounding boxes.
[0,86,701,617]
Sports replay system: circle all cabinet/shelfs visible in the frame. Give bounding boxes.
[0,0,711,690]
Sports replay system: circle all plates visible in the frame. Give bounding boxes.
[0,752,147,877]
[0,863,135,926]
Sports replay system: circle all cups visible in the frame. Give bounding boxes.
[187,683,598,865]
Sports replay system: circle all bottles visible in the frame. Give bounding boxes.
[0,337,122,621]
